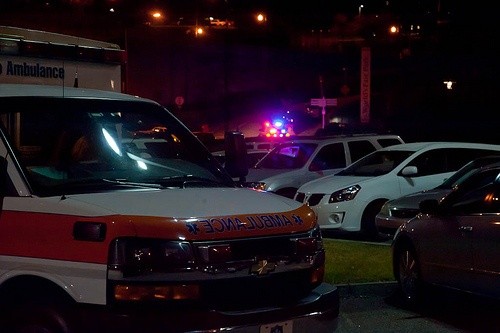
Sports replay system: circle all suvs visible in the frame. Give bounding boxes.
[239,134,406,200]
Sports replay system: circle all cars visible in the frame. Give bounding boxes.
[376,155,500,239]
[391,163,500,315]
[212,150,295,174]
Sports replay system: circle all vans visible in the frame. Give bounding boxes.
[1,84,341,332]
[294,142,500,241]
